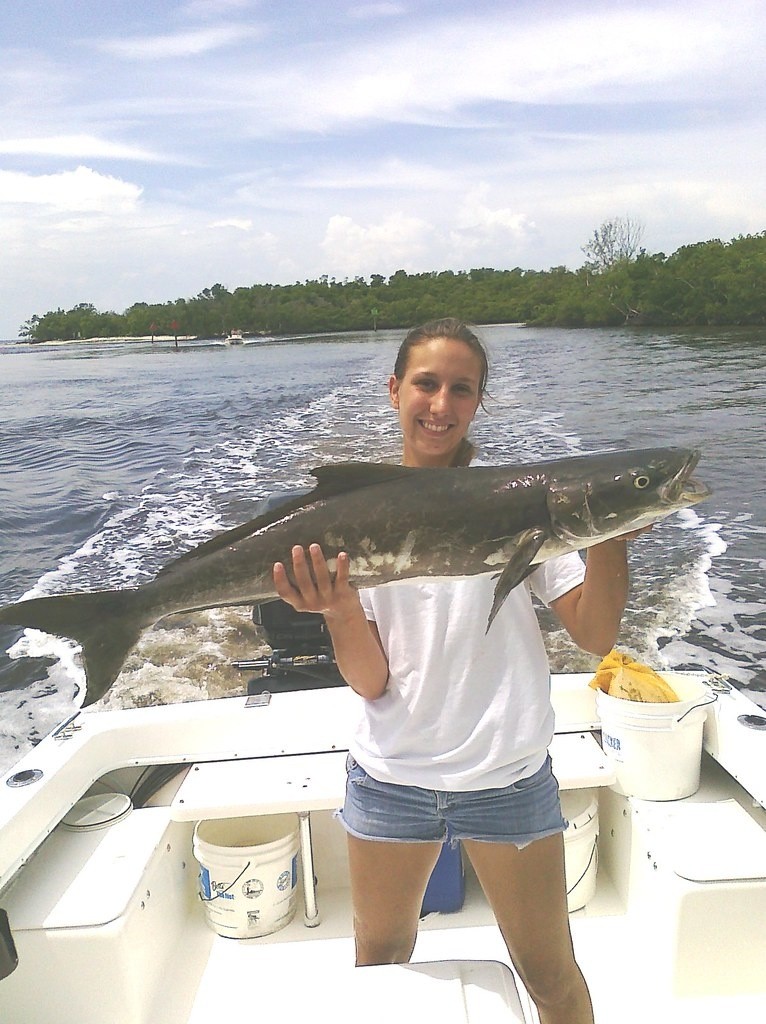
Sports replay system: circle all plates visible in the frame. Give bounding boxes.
[57,792,134,830]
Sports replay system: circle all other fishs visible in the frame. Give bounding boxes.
[1,446,715,707]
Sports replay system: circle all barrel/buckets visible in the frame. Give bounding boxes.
[538,785,602,915]
[192,811,299,940]
[593,669,719,804]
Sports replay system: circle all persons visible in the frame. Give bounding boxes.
[272,317,654,1024]
